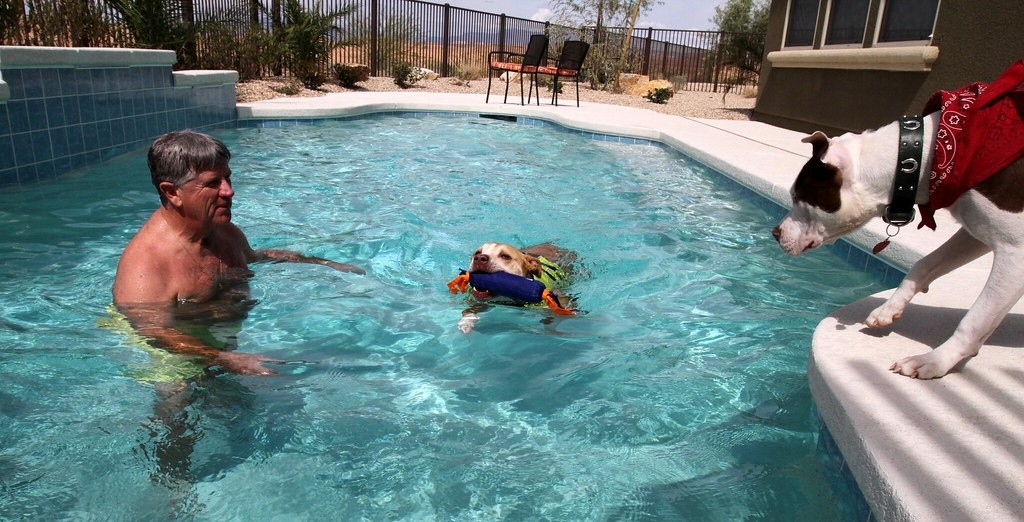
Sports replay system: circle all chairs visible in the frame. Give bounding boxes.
[486,34,549,106]
[528,40,591,107]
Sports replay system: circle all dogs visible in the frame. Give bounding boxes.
[770,53,1024,379]
[460,240,594,337]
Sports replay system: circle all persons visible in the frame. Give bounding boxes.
[112,132,260,304]
